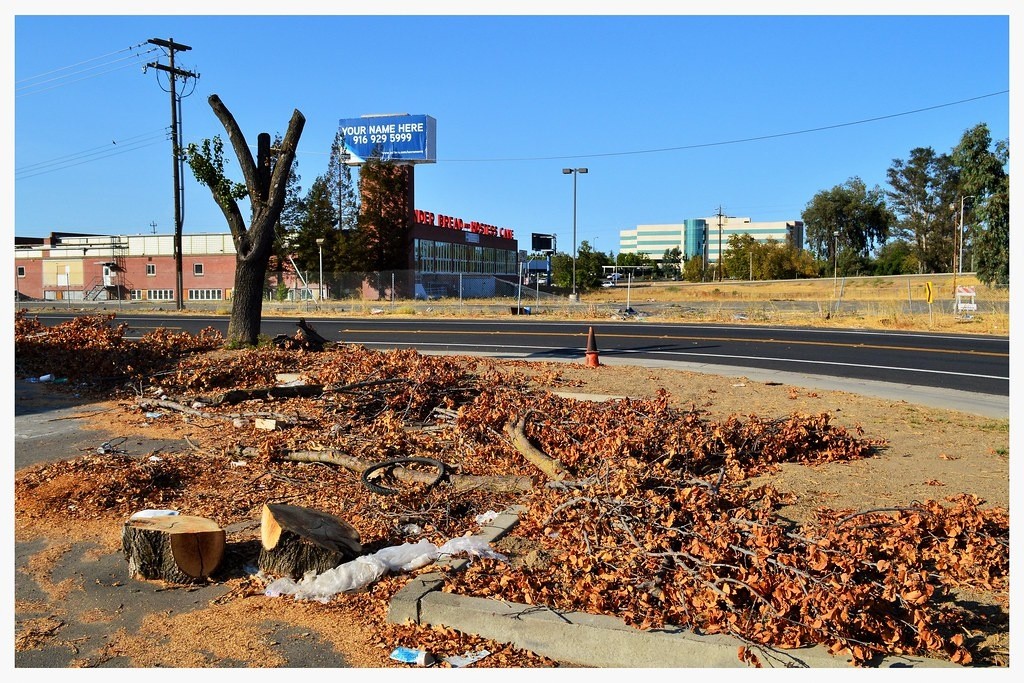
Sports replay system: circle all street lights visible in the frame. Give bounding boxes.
[949,196,976,297]
[316,238,324,301]
[833,231,840,297]
[563,167,587,302]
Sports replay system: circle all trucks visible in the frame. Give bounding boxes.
[612,273,620,280]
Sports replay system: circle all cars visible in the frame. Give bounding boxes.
[538,278,547,285]
[601,281,614,288]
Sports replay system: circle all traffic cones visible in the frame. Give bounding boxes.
[585,327,600,368]
